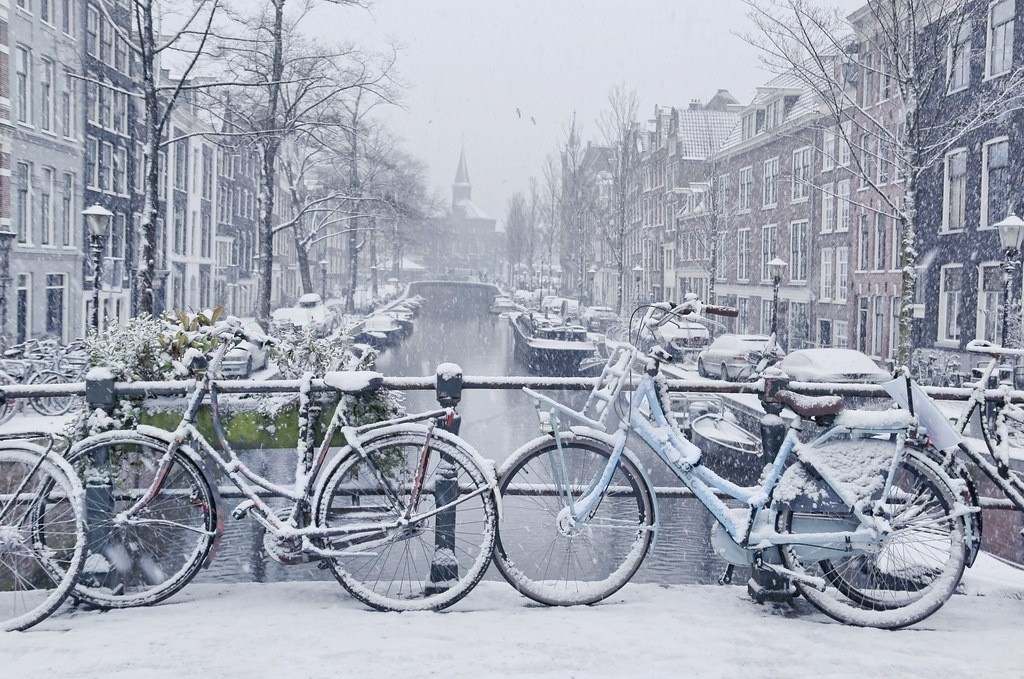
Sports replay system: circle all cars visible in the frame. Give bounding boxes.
[586,312,623,333]
[646,322,710,364]
[218,306,333,380]
[631,315,662,352]
[513,290,566,314]
[580,307,613,327]
[558,300,583,319]
[749,348,896,411]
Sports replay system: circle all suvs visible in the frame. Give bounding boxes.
[697,334,786,382]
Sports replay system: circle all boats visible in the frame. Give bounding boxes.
[507,312,597,376]
[360,295,426,344]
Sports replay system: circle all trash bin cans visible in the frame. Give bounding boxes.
[970,368,998,439]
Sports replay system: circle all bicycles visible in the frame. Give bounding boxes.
[0,316,504,632]
[484,293,1024,629]
[736,349,778,382]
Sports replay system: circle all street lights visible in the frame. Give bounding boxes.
[587,269,596,306]
[319,260,329,305]
[556,270,563,297]
[80,201,115,335]
[538,254,544,312]
[767,256,788,365]
[993,211,1024,378]
[545,247,552,318]
[633,264,644,317]
